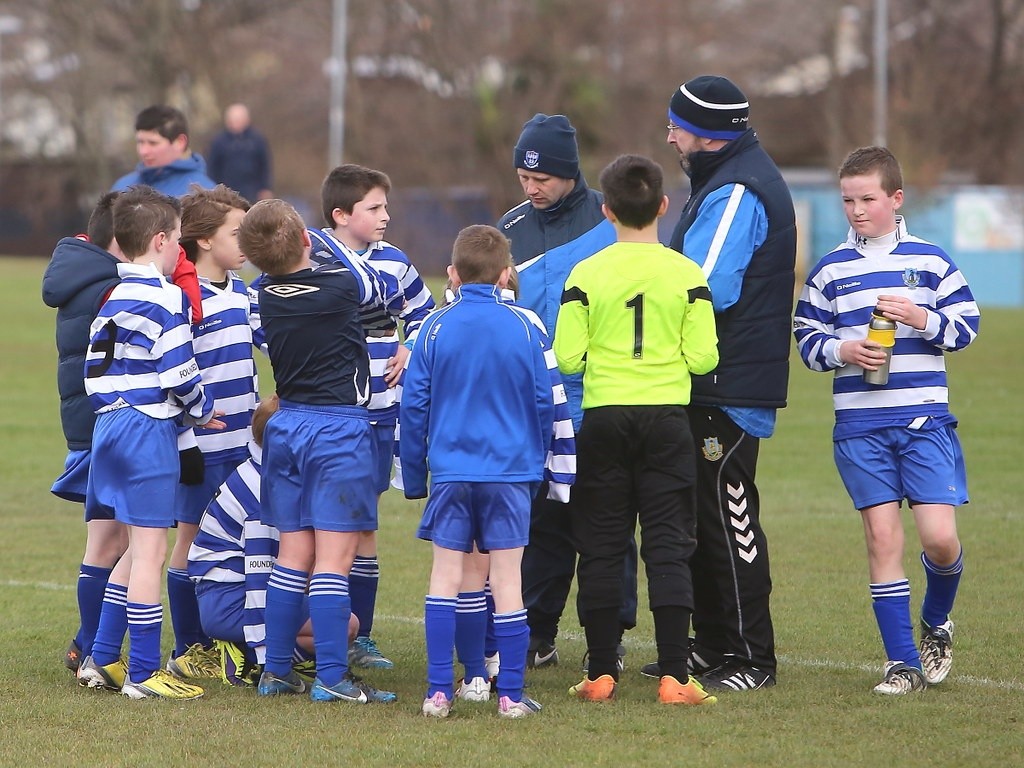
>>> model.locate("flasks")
[862,307,897,386]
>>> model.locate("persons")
[187,396,361,693]
[168,183,315,678]
[794,146,979,693]
[238,200,395,700]
[204,104,272,202]
[639,76,798,692]
[77,185,225,699]
[111,104,216,198]
[43,192,130,670]
[402,222,577,718]
[496,113,624,669]
[246,166,435,666]
[551,155,718,703]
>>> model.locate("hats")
[668,76,750,140]
[512,113,580,180]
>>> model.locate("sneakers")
[483,650,501,681]
[567,673,617,703]
[581,645,627,677]
[454,677,492,702]
[64,637,398,703]
[526,642,558,669]
[698,667,777,691]
[423,691,453,718]
[873,658,928,696]
[642,634,722,677]
[917,613,954,685]
[497,696,542,720]
[658,673,718,706]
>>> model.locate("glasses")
[667,125,679,133]
